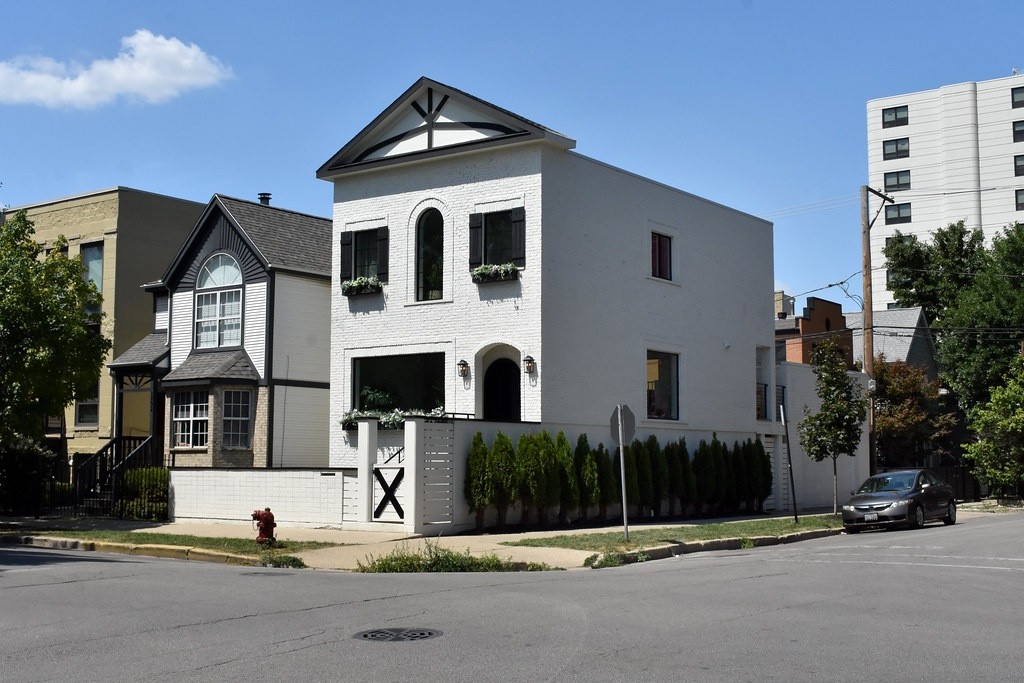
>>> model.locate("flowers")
[338,406,448,431]
[341,275,382,296]
[470,262,517,283]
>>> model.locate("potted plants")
[425,261,443,299]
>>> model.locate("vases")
[472,270,517,282]
[342,285,381,295]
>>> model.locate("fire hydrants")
[251,507,276,544]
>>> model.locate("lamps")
[457,359,468,377]
[523,356,534,373]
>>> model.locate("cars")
[841,469,956,534]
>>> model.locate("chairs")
[908,479,914,487]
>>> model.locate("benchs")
[894,479,927,489]
